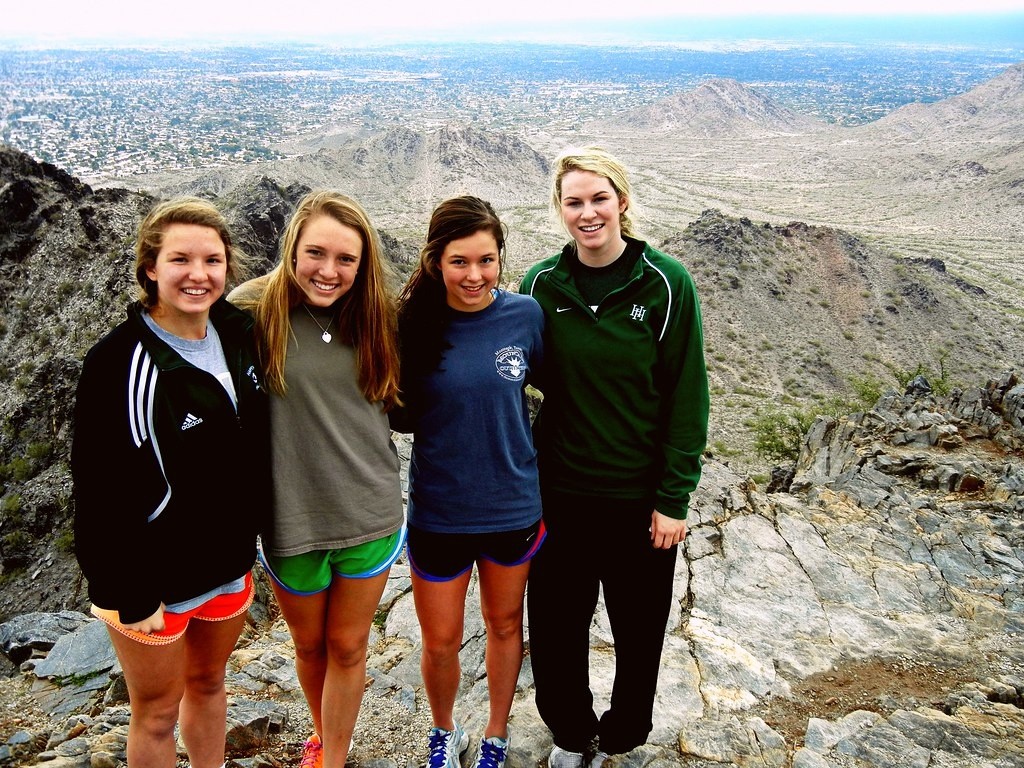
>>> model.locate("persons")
[518,153,709,768]
[70,200,273,768]
[224,186,409,768]
[386,193,547,768]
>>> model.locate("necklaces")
[301,302,336,344]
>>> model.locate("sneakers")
[426,717,470,768]
[472,731,509,768]
[299,732,323,768]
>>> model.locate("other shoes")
[550,744,584,768]
[592,752,609,768]
